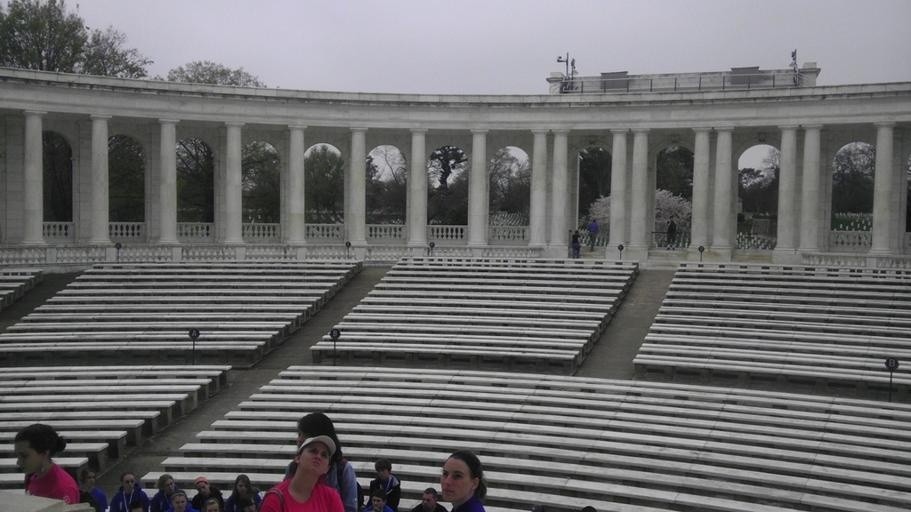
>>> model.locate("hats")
[192,476,208,485]
[297,435,336,457]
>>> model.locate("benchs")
[1,256,911,510]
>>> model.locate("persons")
[571,230,582,258]
[73,466,262,511]
[10,423,81,505]
[667,217,676,250]
[587,219,599,252]
[438,451,491,512]
[411,488,447,511]
[357,463,401,511]
[259,435,345,511]
[282,411,361,512]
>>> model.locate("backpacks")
[288,458,364,508]
